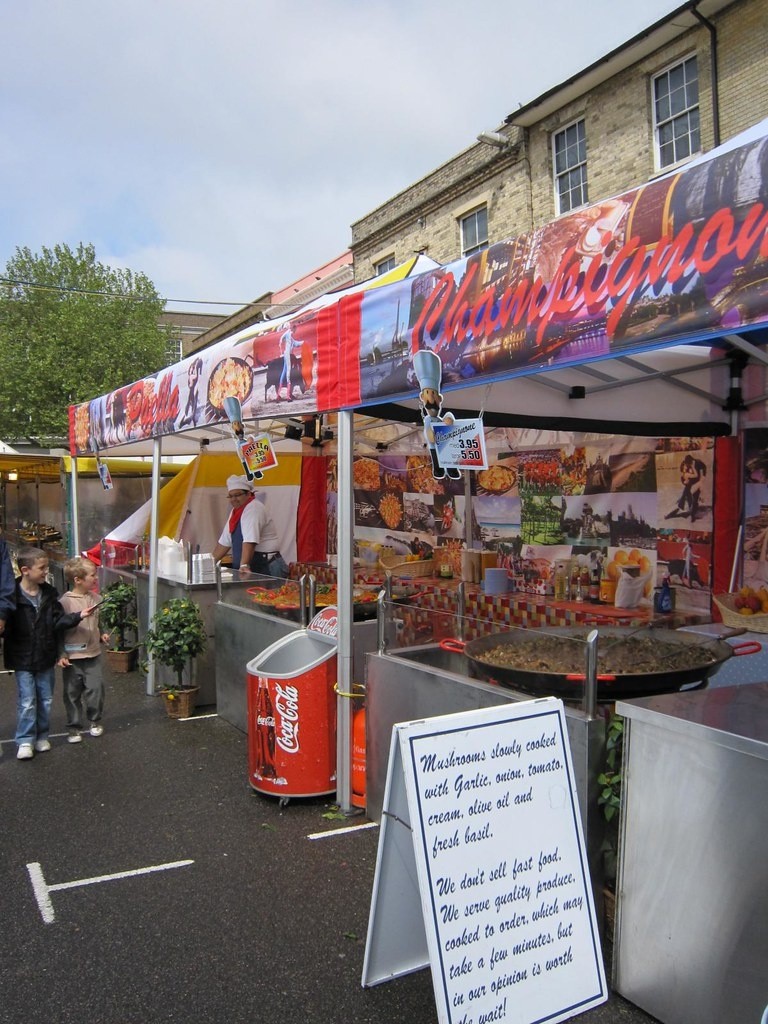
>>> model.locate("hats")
[227,474,254,492]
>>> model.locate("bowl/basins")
[477,465,517,493]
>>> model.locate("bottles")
[566,561,603,605]
[555,564,567,601]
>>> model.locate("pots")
[440,626,762,700]
[246,581,426,623]
[207,355,256,415]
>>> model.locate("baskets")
[378,555,436,578]
[711,588,768,633]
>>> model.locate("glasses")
[227,491,248,500]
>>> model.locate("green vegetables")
[313,585,330,595]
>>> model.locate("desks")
[291,560,712,648]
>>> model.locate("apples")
[735,596,762,612]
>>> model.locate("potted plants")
[147,597,207,718]
[596,713,625,937]
[99,575,138,673]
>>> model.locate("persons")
[665,455,708,522]
[0,538,18,634]
[518,448,588,494]
[3,546,98,760]
[9,483,39,526]
[212,475,290,589]
[56,557,111,743]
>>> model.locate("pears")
[738,583,768,615]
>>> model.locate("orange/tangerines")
[606,549,653,598]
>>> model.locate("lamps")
[8,468,20,483]
[476,131,508,150]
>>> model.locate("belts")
[268,554,279,564]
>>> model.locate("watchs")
[241,564,251,568]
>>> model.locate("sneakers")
[67,735,82,743]
[89,724,103,737]
[17,743,33,759]
[35,740,50,751]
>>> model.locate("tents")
[65,252,726,806]
[342,118,768,812]
